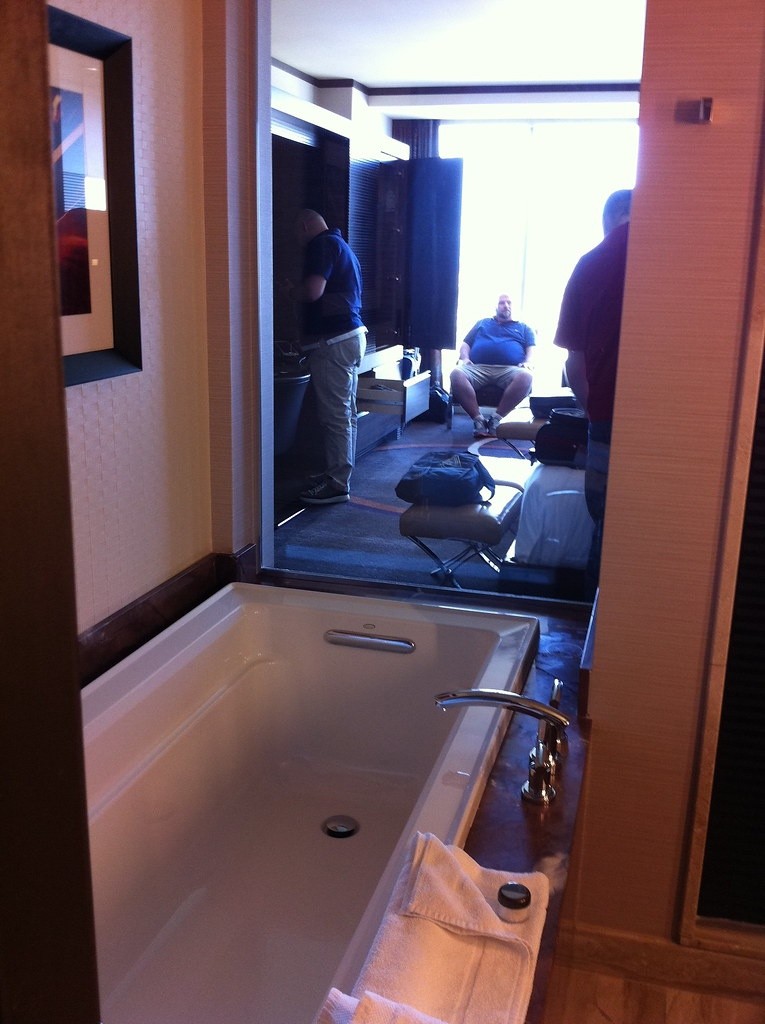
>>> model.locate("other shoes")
[472,415,488,436]
[487,416,499,437]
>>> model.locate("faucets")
[431,677,571,810]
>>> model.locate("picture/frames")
[47,4,144,387]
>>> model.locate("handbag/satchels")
[395,451,495,506]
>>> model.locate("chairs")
[450,383,505,425]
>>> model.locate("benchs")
[395,450,533,595]
[496,386,573,458]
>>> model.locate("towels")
[318,830,551,1023]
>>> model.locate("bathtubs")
[82,580,541,1023]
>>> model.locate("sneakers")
[307,477,333,487]
[299,480,351,504]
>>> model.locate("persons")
[450,294,536,438]
[553,190,633,527]
[283,209,368,504]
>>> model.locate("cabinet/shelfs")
[278,343,430,500]
